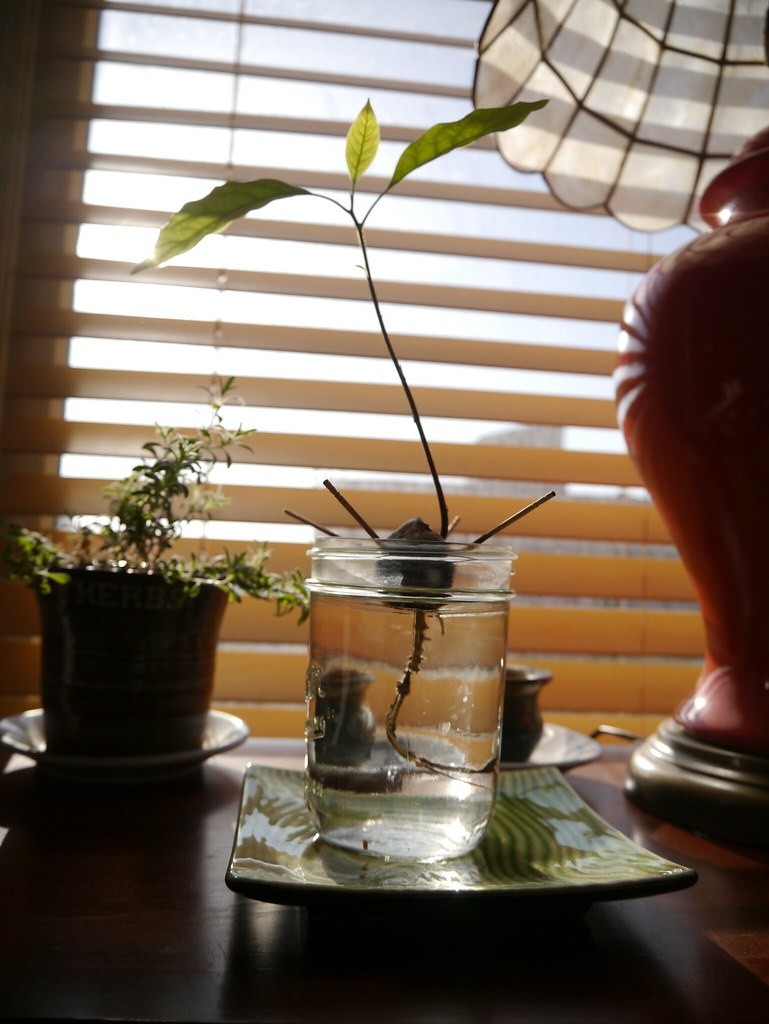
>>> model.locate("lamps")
[473,1,769,751]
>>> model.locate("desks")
[0,736,769,1023]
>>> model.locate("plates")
[2,705,249,784]
[500,723,605,772]
[223,764,700,948]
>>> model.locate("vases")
[304,538,521,862]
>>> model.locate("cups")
[304,535,513,860]
[497,666,554,762]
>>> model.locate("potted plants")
[131,99,552,788]
[1,376,312,784]
